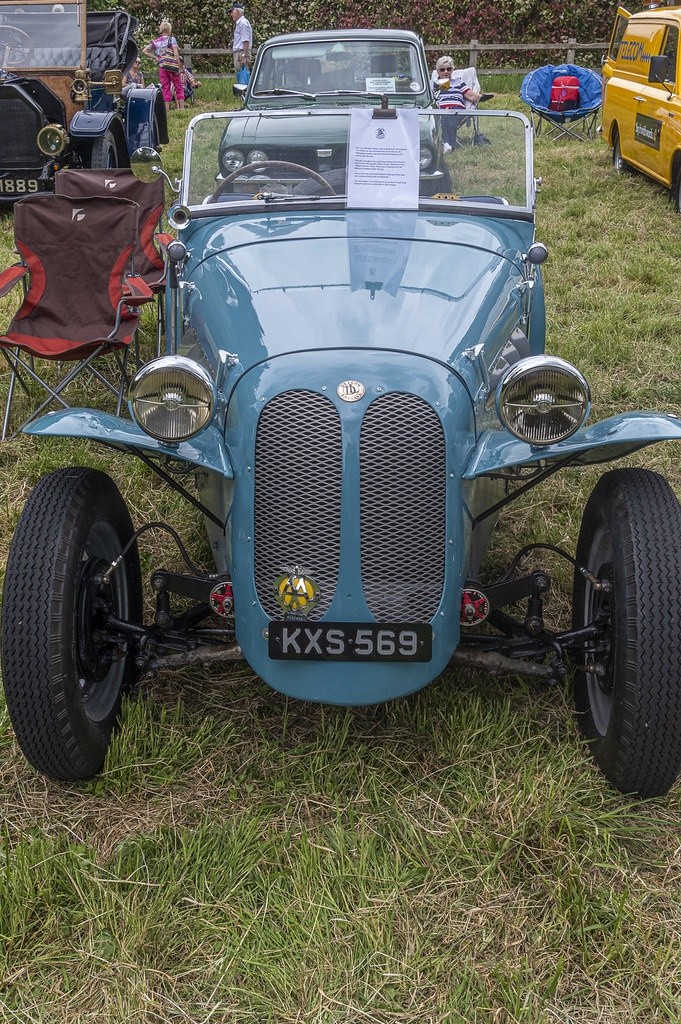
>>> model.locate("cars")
[0,108,681,801]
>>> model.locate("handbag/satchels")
[473,134,492,145]
[158,50,183,73]
[236,65,250,85]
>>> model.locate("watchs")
[242,54,246,57]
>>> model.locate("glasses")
[135,61,141,64]
[440,67,451,72]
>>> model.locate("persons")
[142,22,185,110]
[171,56,202,103]
[120,57,145,89]
[227,3,253,111]
[433,56,494,155]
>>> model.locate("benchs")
[0,46,120,80]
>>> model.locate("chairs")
[292,59,321,85]
[371,53,397,78]
[520,63,604,143]
[154,67,200,105]
[428,67,494,146]
[0,168,174,444]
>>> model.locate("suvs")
[0,0,171,215]
[216,28,451,199]
[599,5,681,210]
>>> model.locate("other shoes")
[442,143,452,156]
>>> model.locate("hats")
[179,56,184,63]
[228,3,242,12]
